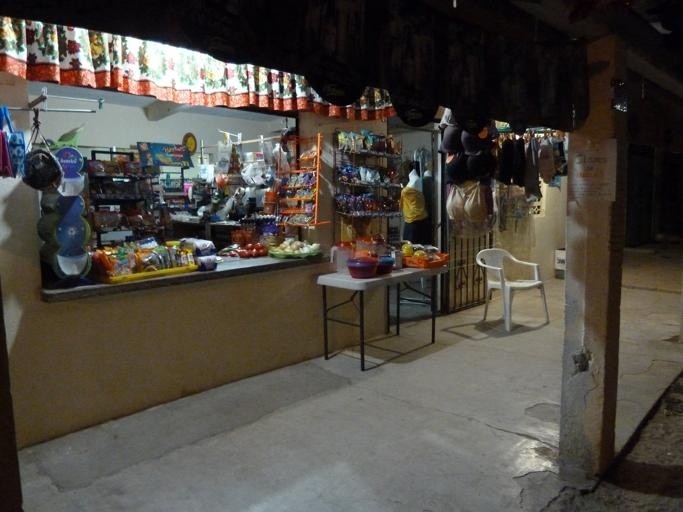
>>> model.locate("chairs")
[476,248,550,332]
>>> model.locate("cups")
[329,241,352,275]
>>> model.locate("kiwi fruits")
[279,239,312,250]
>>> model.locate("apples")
[228,242,268,257]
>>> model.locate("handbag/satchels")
[0,107,15,177]
[3,106,25,178]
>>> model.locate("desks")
[317,265,449,372]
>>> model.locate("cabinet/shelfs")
[85,135,299,251]
[330,128,403,243]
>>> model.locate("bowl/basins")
[347,257,394,278]
